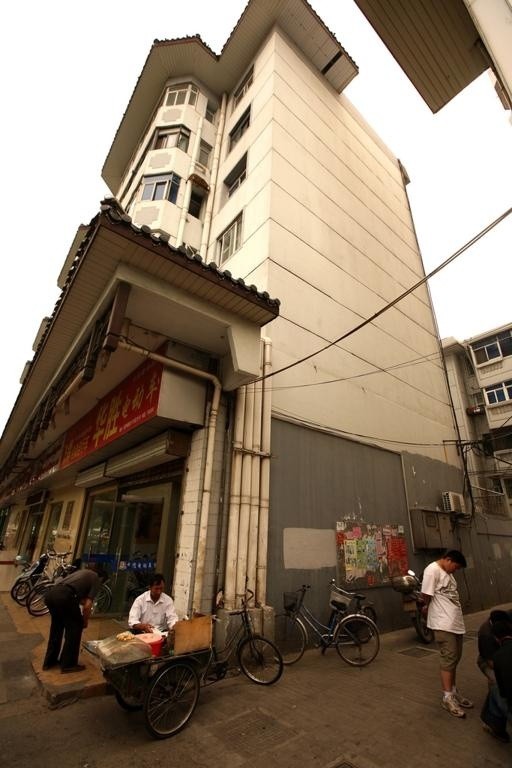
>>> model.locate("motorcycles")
[391,569,434,644]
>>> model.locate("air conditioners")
[442,492,466,513]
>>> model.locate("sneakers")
[42,656,86,675]
[440,692,466,718]
[453,685,473,708]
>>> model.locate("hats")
[445,549,467,569]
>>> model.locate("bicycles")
[273,578,380,667]
[11,543,112,618]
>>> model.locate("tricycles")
[81,589,284,740]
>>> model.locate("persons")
[127,573,180,634]
[42,564,108,674]
[420,549,474,719]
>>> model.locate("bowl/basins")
[147,638,165,657]
